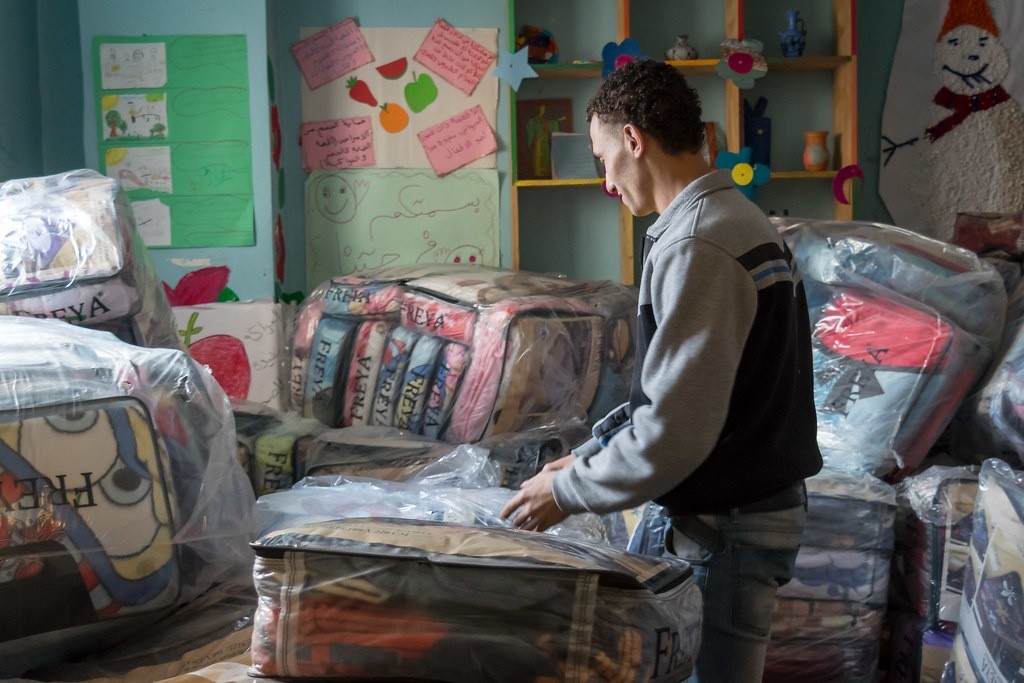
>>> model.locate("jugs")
[781,9,807,57]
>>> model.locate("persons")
[500,61,824,683]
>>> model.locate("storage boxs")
[168,300,295,412]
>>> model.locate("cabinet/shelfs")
[505,0,858,289]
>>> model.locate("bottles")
[802,130,830,171]
